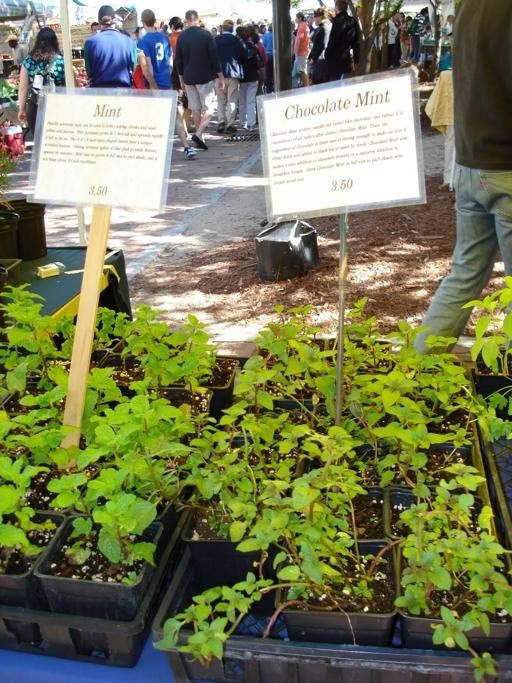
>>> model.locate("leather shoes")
[191,134,208,149]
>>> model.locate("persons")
[3,34,29,67]
[89,21,100,36]
[138,0,406,161]
[83,4,138,87]
[18,26,64,143]
[406,0,454,64]
[406,0,511,355]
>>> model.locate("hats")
[99,5,116,21]
[222,19,233,25]
[169,16,181,24]
[5,34,18,42]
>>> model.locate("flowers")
[0,120,25,211]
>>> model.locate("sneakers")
[238,122,247,128]
[226,126,236,134]
[247,124,255,131]
[185,147,197,159]
[217,123,225,132]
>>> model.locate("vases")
[0,198,46,259]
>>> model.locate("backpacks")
[30,57,57,106]
[406,17,423,33]
